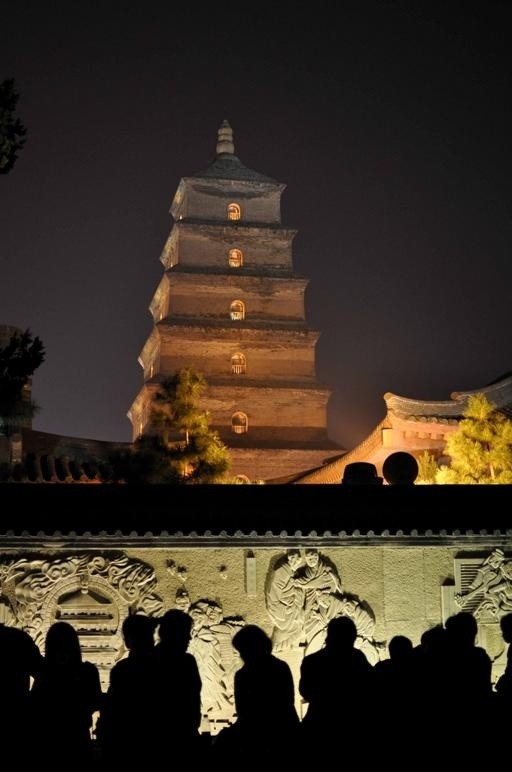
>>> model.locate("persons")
[452,547,512,644]
[1,610,512,771]
[159,547,383,713]
